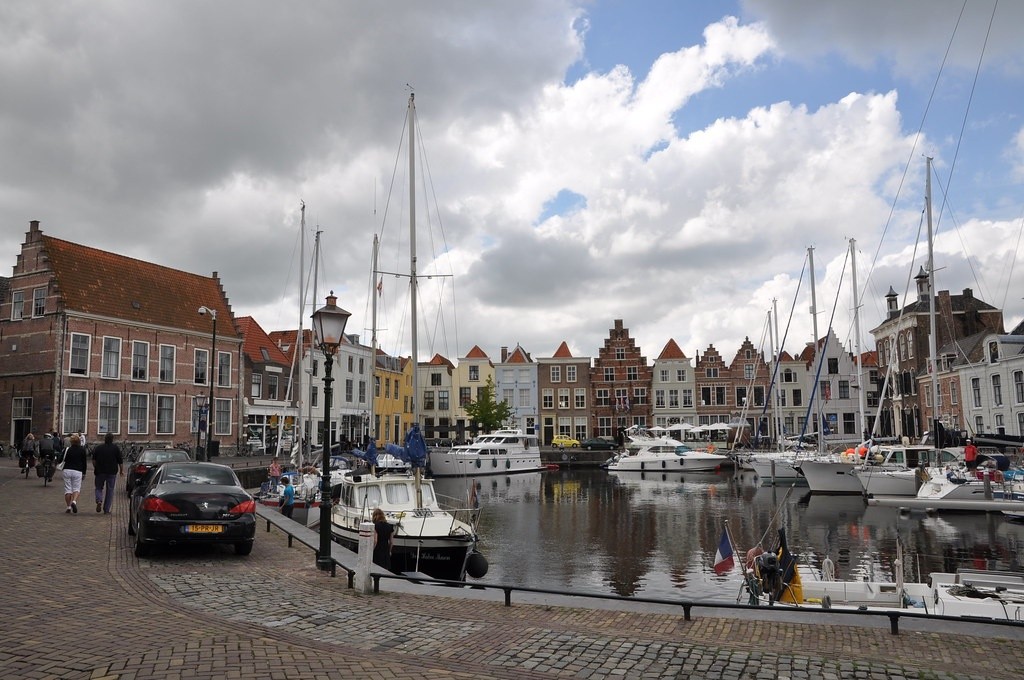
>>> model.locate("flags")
[615,395,631,412]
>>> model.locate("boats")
[913,452,1024,499]
[604,439,727,472]
[429,424,547,475]
[745,566,1024,625]
[992,471,1024,518]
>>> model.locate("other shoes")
[21,469,25,473]
[96,502,102,512]
[66,509,71,513]
[71,502,78,513]
[48,479,52,482]
[104,511,111,514]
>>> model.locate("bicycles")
[225,442,249,457]
[36,454,56,487]
[86,439,194,461]
[25,458,30,479]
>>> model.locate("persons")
[92,433,124,514]
[269,457,282,494]
[964,439,978,471]
[372,509,394,571]
[280,477,294,520]
[19,430,86,482]
[552,442,564,452]
[57,435,87,514]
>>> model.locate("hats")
[44,433,51,437]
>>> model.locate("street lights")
[309,290,352,571]
[194,391,207,462]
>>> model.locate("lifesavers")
[708,446,714,453]
[994,470,1003,483]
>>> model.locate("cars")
[125,448,191,497]
[550,435,580,449]
[128,461,255,558]
[581,437,620,450]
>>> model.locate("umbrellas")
[624,422,732,433]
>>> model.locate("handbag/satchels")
[56,462,65,471]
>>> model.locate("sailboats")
[793,237,891,494]
[726,245,844,480]
[331,92,483,575]
[249,203,352,529]
[853,154,1010,497]
